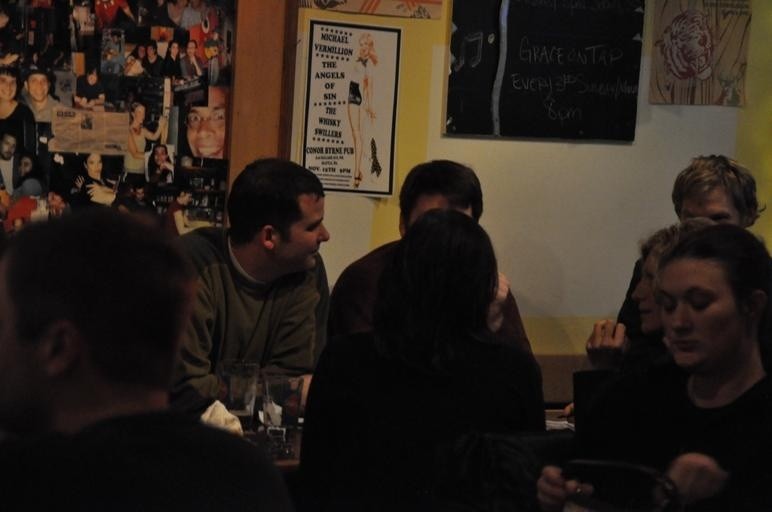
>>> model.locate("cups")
[264,374,305,449]
[227,360,260,429]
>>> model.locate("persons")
[344,30,379,187]
[291,206,546,512]
[671,145,762,236]
[2,193,280,508]
[0,0,233,240]
[568,225,677,452]
[532,223,772,512]
[146,155,332,464]
[322,154,529,344]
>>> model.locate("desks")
[278,415,578,473]
